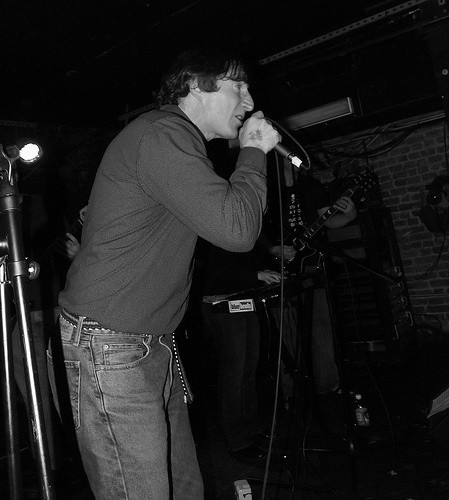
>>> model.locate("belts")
[60,306,194,403]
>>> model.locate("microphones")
[274,143,306,169]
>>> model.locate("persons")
[260,141,358,410]
[59,47,282,500]
[420,175,449,235]
[0,186,89,469]
[198,237,264,461]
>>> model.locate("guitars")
[247,169,365,290]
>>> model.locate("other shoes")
[50,451,60,470]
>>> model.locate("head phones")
[427,176,449,205]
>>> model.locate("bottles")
[352,393,368,427]
